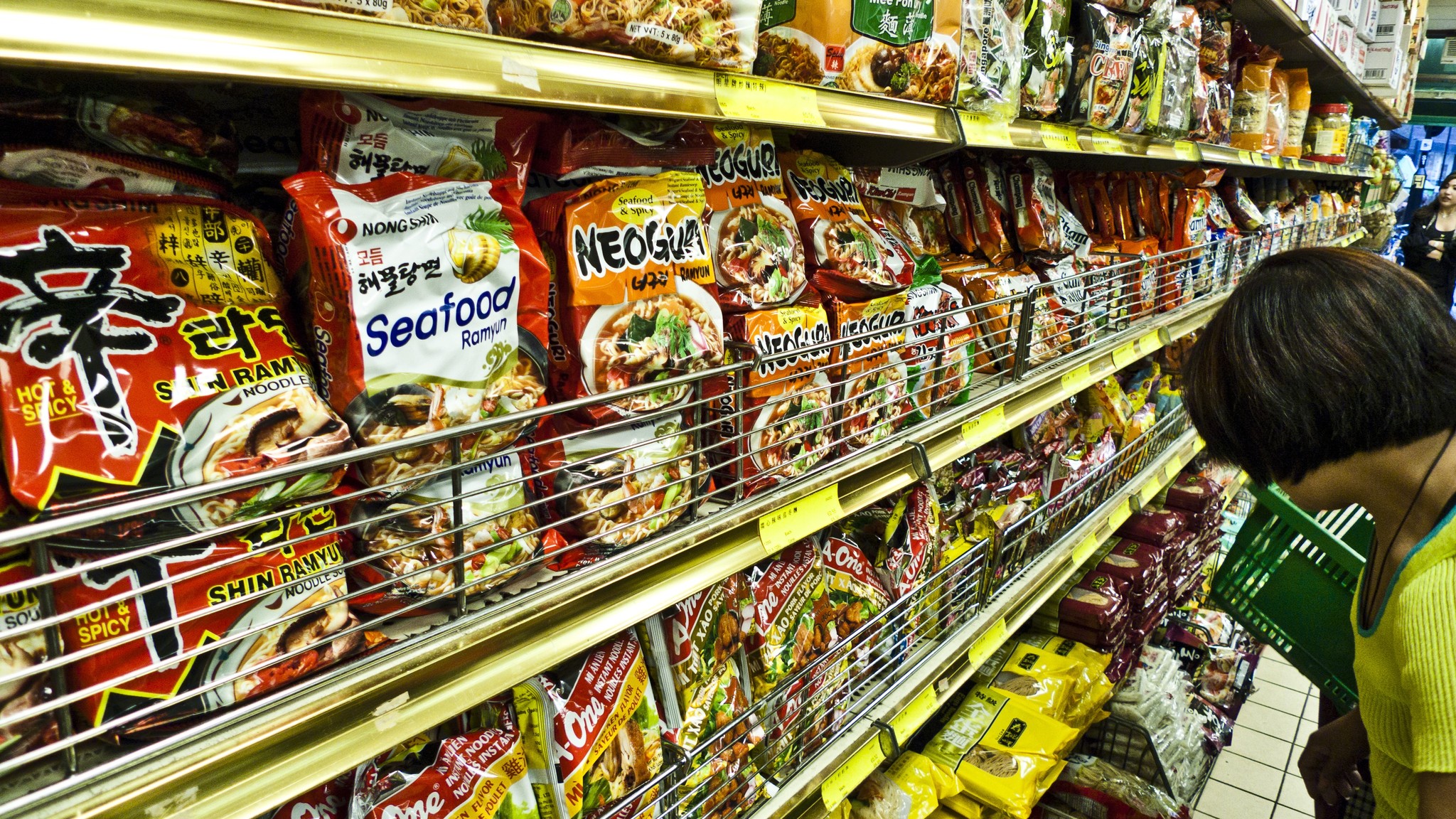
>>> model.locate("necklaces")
[1437,207,1456,218]
[1361,432,1454,629]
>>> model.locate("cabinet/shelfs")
[0,0,1426,818]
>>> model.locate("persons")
[1177,245,1456,819]
[1402,171,1456,316]
